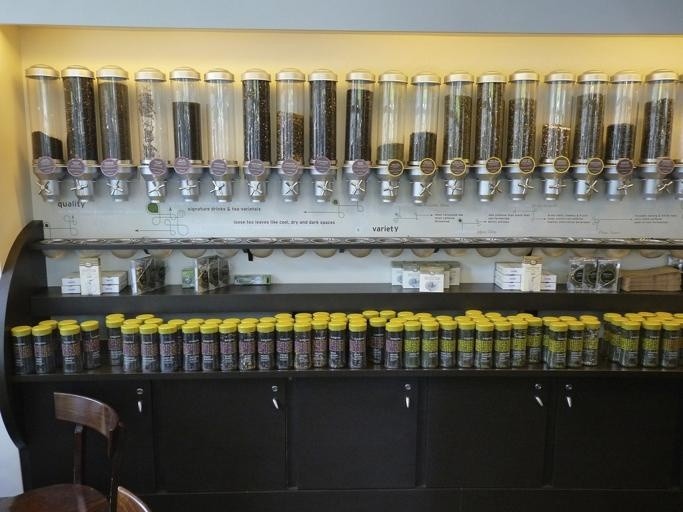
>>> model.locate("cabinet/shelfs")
[423,371,683,493]
[23,231,682,316]
[289,376,425,491]
[153,378,289,490]
[12,377,155,489]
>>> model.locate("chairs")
[0,390,124,512]
[115,476,153,512]
